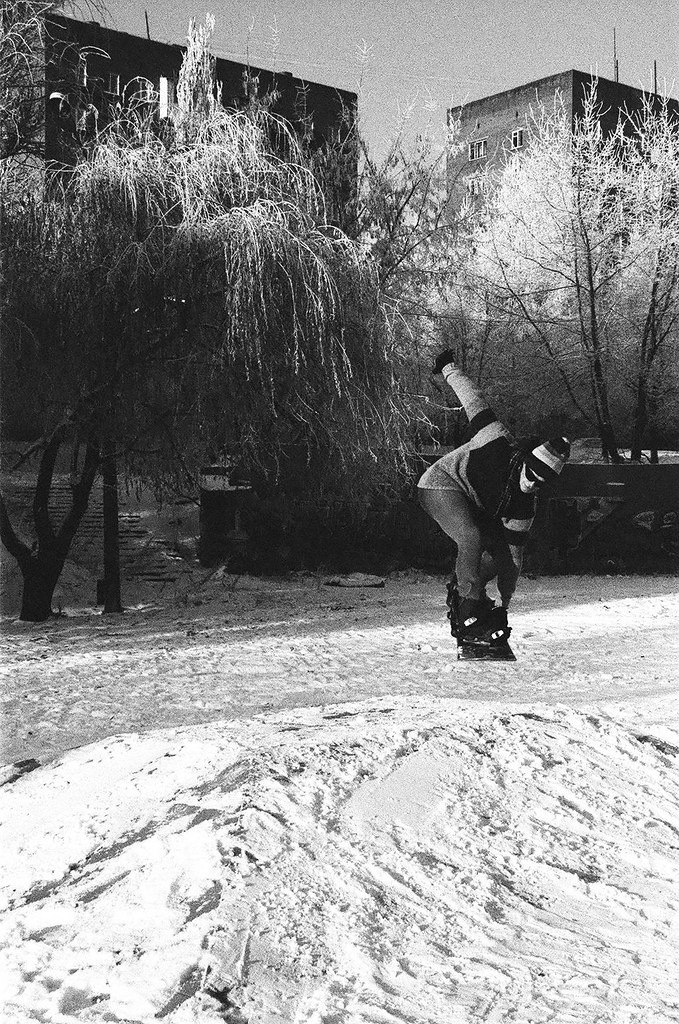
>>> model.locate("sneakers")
[446,576,511,644]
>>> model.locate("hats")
[527,437,571,482]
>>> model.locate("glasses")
[525,467,545,488]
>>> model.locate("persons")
[416,349,571,659]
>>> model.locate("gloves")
[432,349,455,374]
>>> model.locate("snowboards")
[446,581,518,660]
